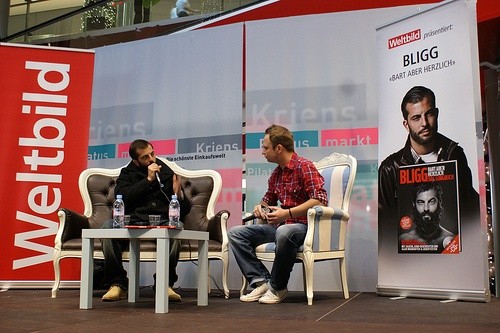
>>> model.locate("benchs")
[51,153,231,299]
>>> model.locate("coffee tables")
[79,228,209,313]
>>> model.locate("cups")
[149,215,161,226]
[124,215,130,225]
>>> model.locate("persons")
[227,124,328,303]
[133,0,150,25]
[378,85,481,234]
[99,139,191,302]
[175,0,199,18]
[400,181,454,249]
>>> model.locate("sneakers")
[101,286,127,300]
[240,281,270,302]
[168,287,181,301]
[259,288,288,304]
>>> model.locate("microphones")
[242,208,271,223]
[155,172,161,184]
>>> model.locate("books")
[397,159,463,254]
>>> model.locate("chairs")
[239,152,358,306]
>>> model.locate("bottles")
[113,195,125,229]
[168,195,180,227]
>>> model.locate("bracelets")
[288,208,293,217]
